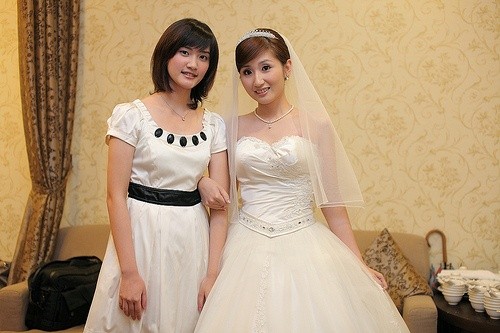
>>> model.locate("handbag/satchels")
[25,255,102,331]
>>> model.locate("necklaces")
[254,104,293,129]
[159,93,192,121]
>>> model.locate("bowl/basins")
[437,277,500,319]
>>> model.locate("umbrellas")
[426,229,454,290]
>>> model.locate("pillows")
[362,228,434,316]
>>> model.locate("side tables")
[432,288,500,333]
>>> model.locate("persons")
[82,18,229,333]
[196,28,410,333]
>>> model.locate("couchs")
[0,225,438,333]
